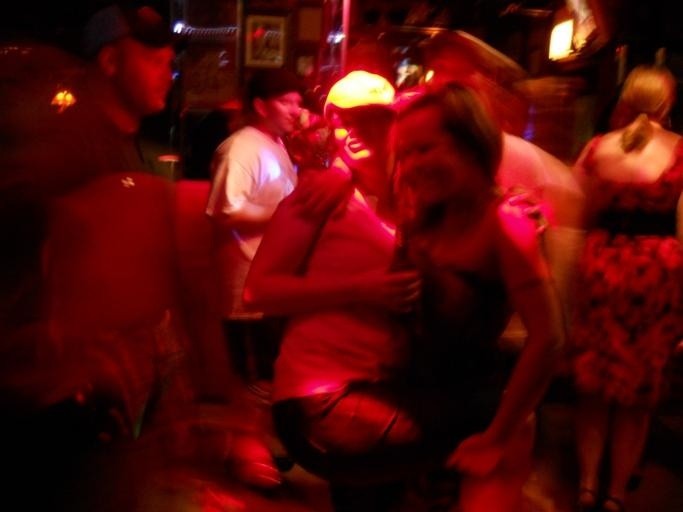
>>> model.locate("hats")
[318,70,396,124]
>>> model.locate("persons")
[1,1,683,511]
